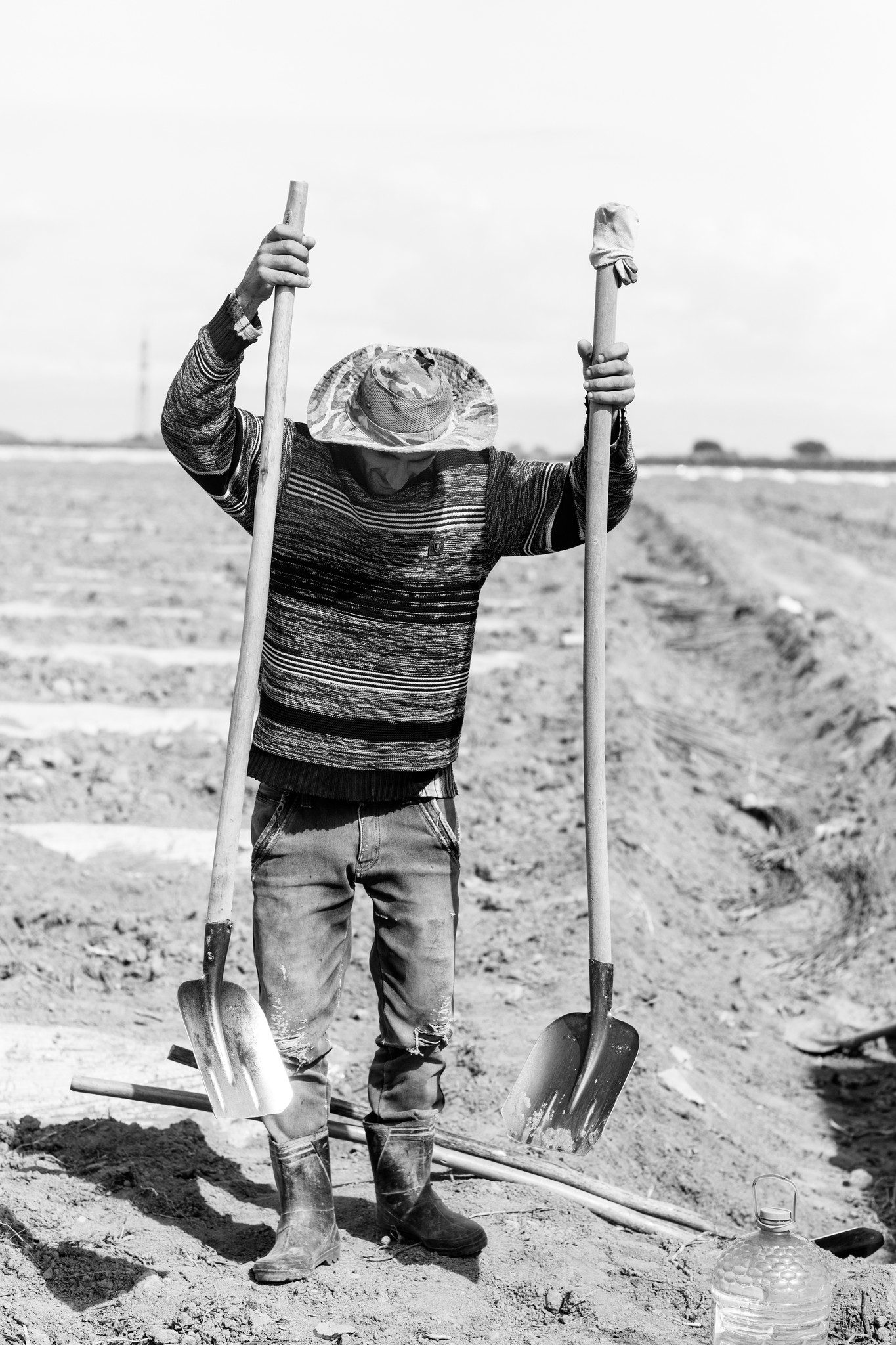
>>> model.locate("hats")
[309,344,499,454]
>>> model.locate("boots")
[252,1129,341,1280]
[363,1109,486,1256]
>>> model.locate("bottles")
[709,1173,833,1344]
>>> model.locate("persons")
[159,222,638,1284]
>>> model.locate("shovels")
[177,177,296,1123]
[498,203,641,1161]
[68,1043,887,1261]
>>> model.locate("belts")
[258,782,387,812]
